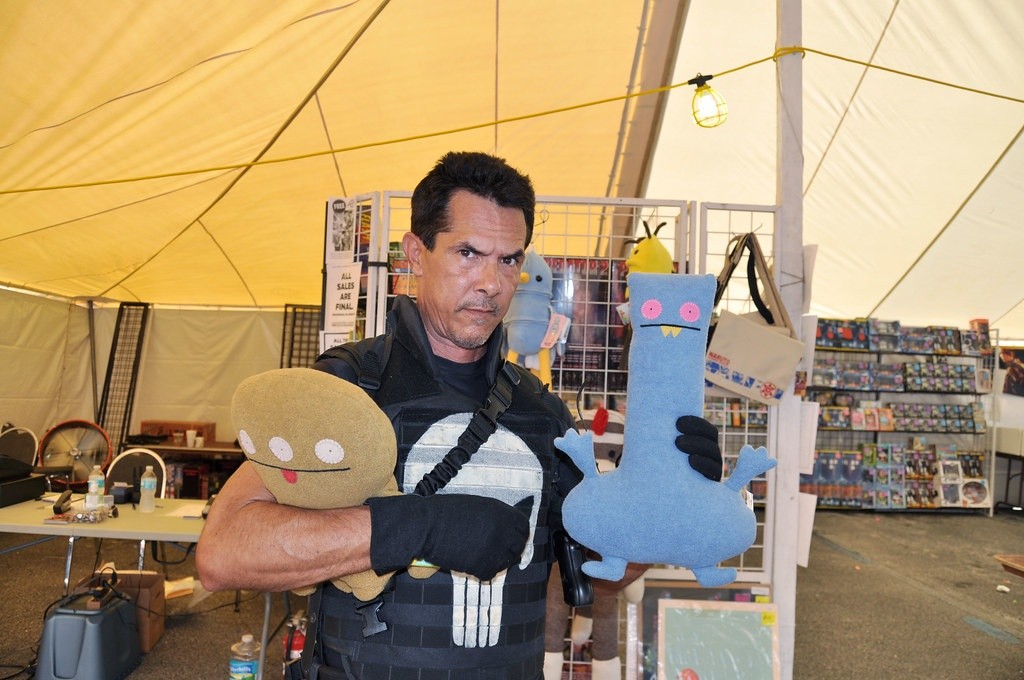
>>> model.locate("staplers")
[53,490,73,514]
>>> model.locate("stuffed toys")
[548,272,777,586]
[231,368,442,602]
[541,400,644,680]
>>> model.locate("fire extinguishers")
[281,610,309,680]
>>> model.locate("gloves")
[362,495,529,581]
[675,414,723,482]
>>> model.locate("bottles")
[139,465,157,513]
[228,632,262,680]
[87,464,106,511]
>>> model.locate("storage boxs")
[74,573,165,653]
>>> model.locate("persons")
[193,146,725,680]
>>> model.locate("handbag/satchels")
[709,232,805,406]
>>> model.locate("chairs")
[61,448,167,598]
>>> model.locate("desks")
[127,438,243,454]
[0,494,209,543]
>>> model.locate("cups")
[186,430,196,448]
[174,433,183,448]
[195,437,204,448]
